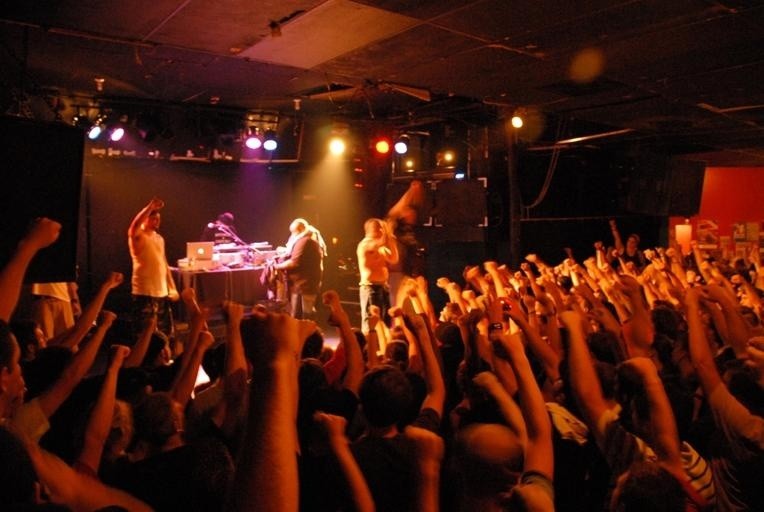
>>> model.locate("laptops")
[177,241,214,263]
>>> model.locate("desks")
[176,258,274,320]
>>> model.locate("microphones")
[207,223,222,229]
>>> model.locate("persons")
[127,196,182,319]
[0,216,764,511]
[389,181,428,308]
